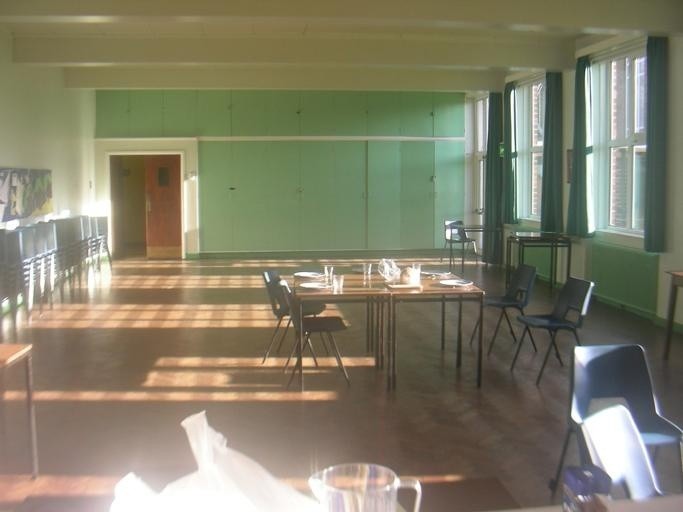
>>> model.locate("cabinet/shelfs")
[197,90,266,138]
[267,141,333,260]
[332,141,401,259]
[93,88,162,138]
[400,140,466,259]
[266,89,332,138]
[331,89,401,138]
[162,89,197,138]
[401,90,466,137]
[197,141,267,259]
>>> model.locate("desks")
[451,224,502,271]
[506,234,571,298]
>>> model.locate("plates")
[300,282,330,289]
[440,279,473,286]
[294,271,322,278]
[421,271,451,276]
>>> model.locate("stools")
[0,342,38,480]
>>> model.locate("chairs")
[550,345,683,500]
[512,276,593,386]
[261,271,328,367]
[469,266,537,355]
[280,280,351,387]
[440,219,477,269]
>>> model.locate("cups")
[413,263,421,273]
[333,275,344,293]
[363,263,372,275]
[309,462,422,508]
[325,265,333,277]
